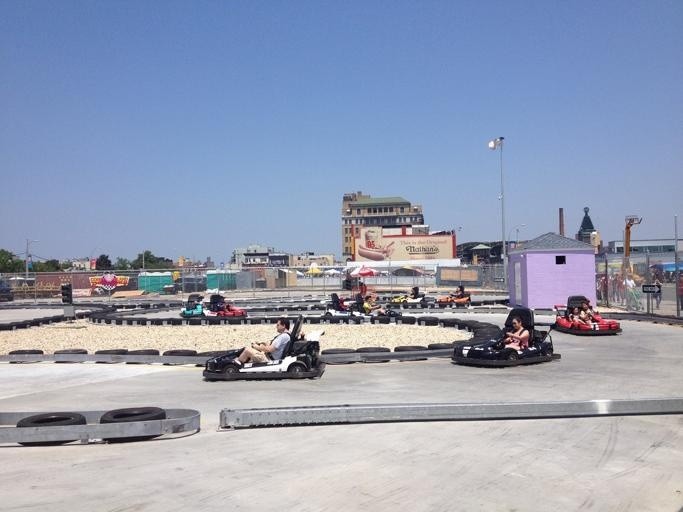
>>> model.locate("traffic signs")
[641,283,660,294]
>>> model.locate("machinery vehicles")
[616,213,645,288]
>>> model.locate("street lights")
[457,225,463,258]
[515,224,527,246]
[488,135,507,286]
[25,239,40,280]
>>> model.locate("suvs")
[163,277,207,294]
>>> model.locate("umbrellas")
[350,265,376,293]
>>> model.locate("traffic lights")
[61,285,72,304]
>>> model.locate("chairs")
[565,296,592,321]
[331,293,343,311]
[251,315,304,367]
[356,294,366,313]
[210,295,221,312]
[499,308,535,348]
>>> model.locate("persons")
[580,300,594,324]
[234,318,292,368]
[192,300,200,308]
[339,297,348,309]
[450,286,464,297]
[408,287,418,299]
[217,301,229,312]
[596,265,683,311]
[362,295,385,316]
[568,306,585,325]
[503,316,532,350]
